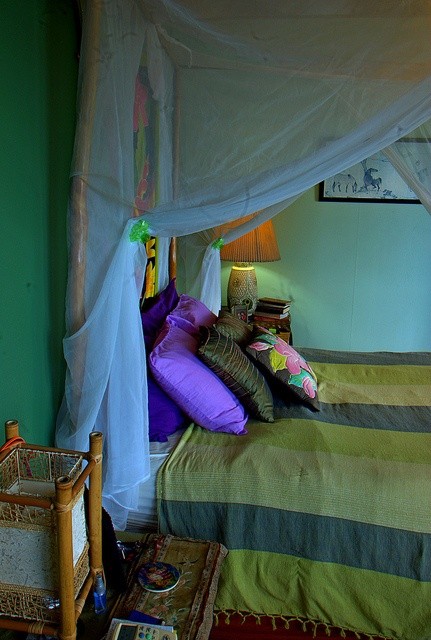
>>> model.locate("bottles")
[94,575,106,615]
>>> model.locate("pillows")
[198,324,276,424]
[149,294,250,437]
[212,309,253,346]
[140,277,190,444]
[245,323,321,413]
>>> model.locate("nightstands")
[222,305,292,345]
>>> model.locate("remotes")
[111,623,178,640]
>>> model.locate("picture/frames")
[317,134,431,205]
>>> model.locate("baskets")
[0,448,90,625]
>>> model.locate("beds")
[62,1,431,640]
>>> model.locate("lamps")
[208,206,282,317]
[0,419,109,640]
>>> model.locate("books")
[253,297,292,322]
